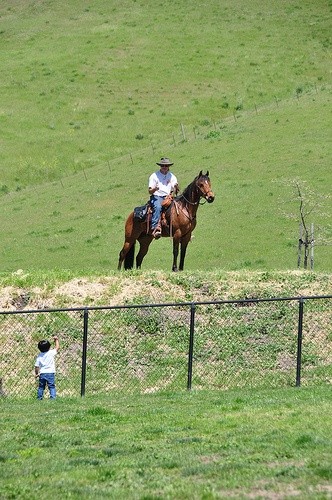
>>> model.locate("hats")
[156,157,174,166]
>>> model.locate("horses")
[118,170,214,271]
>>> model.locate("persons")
[34,335,60,400]
[148,157,178,239]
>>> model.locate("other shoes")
[154,231,162,240]
